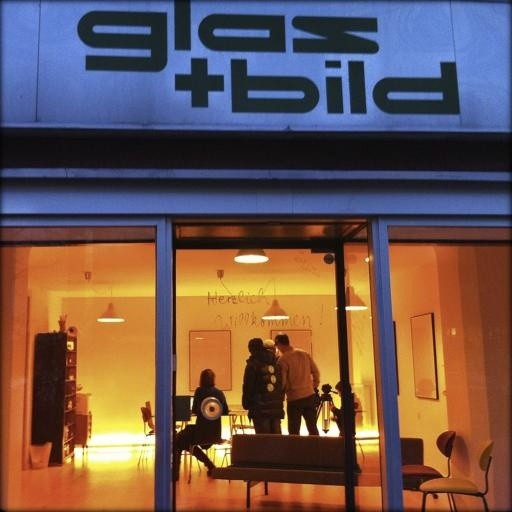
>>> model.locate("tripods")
[317,393,364,457]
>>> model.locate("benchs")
[207,433,360,509]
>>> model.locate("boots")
[193,446,215,474]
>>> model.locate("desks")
[186,408,254,461]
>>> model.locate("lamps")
[216,269,289,320]
[234,249,268,265]
[324,253,369,311]
[84,269,126,323]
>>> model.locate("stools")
[230,423,255,435]
[180,435,232,483]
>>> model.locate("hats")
[274,334,289,344]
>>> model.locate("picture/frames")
[392,320,401,396]
[188,330,233,391]
[410,311,439,400]
[271,329,312,358]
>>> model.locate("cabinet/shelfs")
[74,411,92,452]
[34,331,76,466]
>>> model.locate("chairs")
[145,400,156,465]
[401,430,460,512]
[418,439,495,511]
[138,407,156,465]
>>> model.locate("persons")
[261,340,279,359]
[275,334,319,435]
[242,338,284,434]
[331,381,362,436]
[175,369,228,480]
